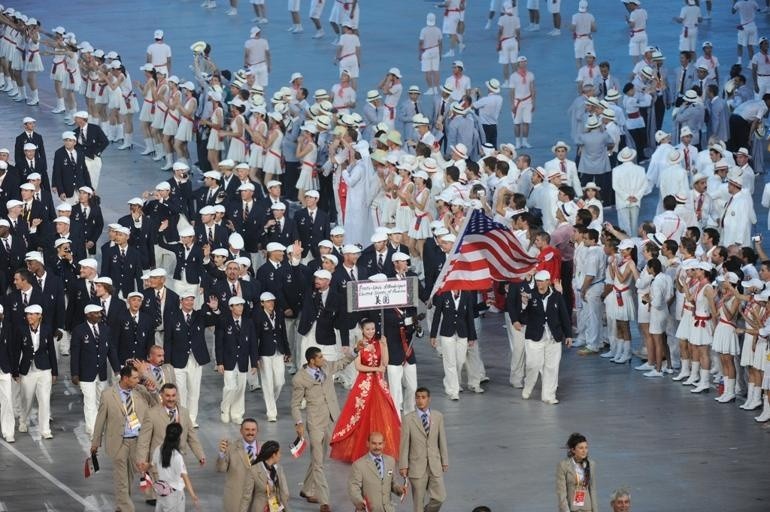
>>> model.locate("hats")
[502,3,515,17]
[453,60,465,72]
[680,40,717,103]
[654,126,752,204]
[3,2,244,121]
[580,0,590,13]
[680,256,769,308]
[342,21,353,30]
[427,12,438,27]
[249,27,259,39]
[534,271,552,282]
[244,182,457,304]
[639,44,666,80]
[757,36,768,45]
[584,50,598,59]
[107,158,242,308]
[246,67,517,181]
[1,130,110,316]
[529,140,602,220]
[615,232,668,250]
[615,146,640,163]
[584,88,623,130]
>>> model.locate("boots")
[610,337,634,366]
[671,358,770,423]
[106,125,171,169]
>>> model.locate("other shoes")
[287,25,303,34]
[442,51,456,59]
[313,28,324,40]
[458,43,466,54]
[251,16,269,25]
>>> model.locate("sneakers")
[2,420,52,443]
[634,360,674,379]
[220,417,244,426]
[446,374,491,401]
[512,380,560,406]
[570,336,610,360]
[266,416,277,422]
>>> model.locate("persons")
[1,1,769,511]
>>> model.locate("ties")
[374,458,383,478]
[314,372,323,380]
[246,448,256,463]
[422,411,429,434]
